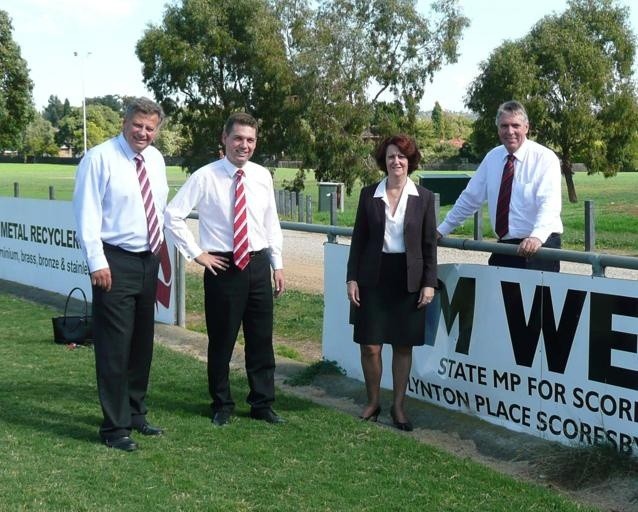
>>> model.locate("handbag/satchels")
[52,316,95,345]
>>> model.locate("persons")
[74,97,170,453]
[164,113,285,428]
[345,134,438,432]
[437,102,564,272]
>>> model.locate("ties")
[135,153,163,256]
[233,170,249,270]
[495,155,515,239]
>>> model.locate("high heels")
[391,406,413,431]
[359,407,380,422]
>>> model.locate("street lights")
[72,50,93,157]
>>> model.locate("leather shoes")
[134,423,163,435]
[213,409,231,425]
[106,436,136,451]
[250,408,289,424]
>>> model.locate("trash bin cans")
[316,181,344,213]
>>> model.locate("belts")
[250,248,271,257]
